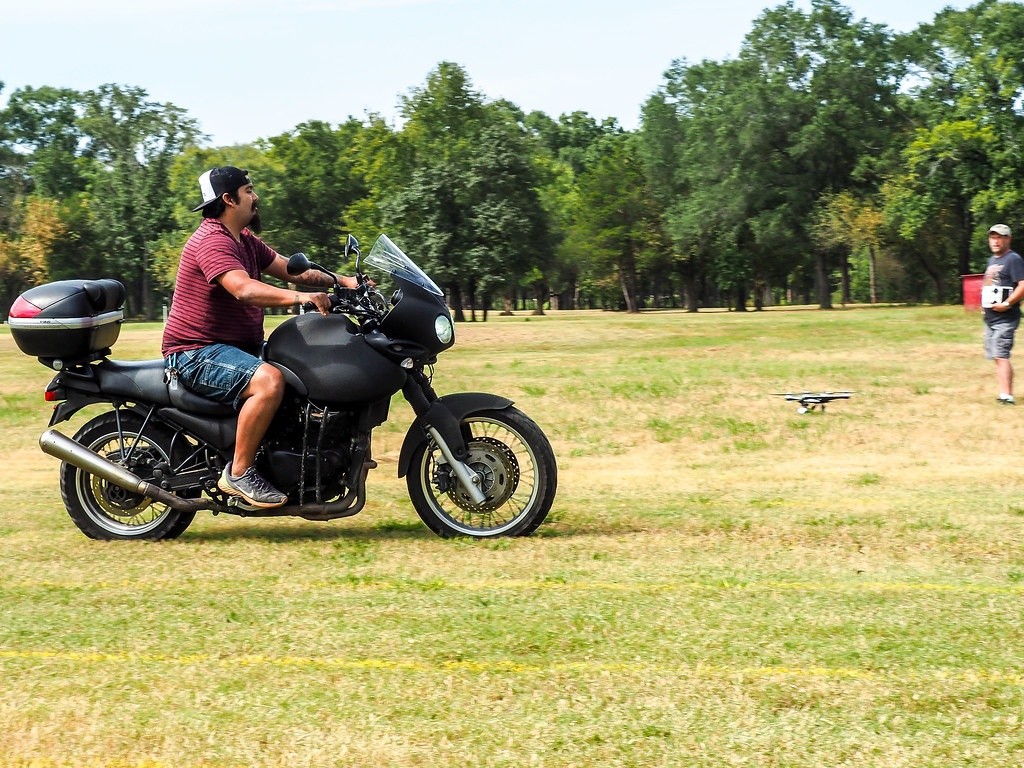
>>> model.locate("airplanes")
[768,390,859,413]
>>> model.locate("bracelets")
[1003,302,1011,308]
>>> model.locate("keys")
[162,367,181,383]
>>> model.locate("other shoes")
[996,393,1015,406]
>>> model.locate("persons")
[160,166,379,509]
[980,224,1024,404]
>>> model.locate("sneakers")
[218,460,288,509]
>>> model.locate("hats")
[987,224,1012,237]
[192,166,251,212]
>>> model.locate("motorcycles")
[6,231,560,543]
